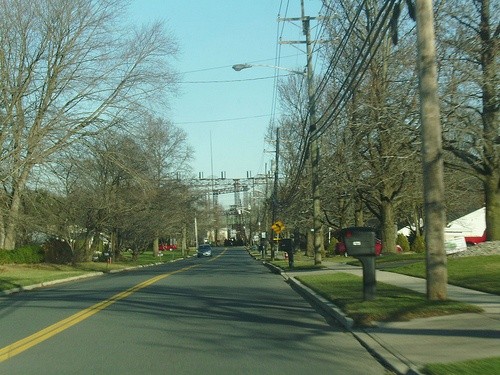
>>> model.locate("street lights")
[232,63,326,266]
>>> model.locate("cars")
[159,242,177,251]
[336,238,404,258]
[196,243,214,257]
